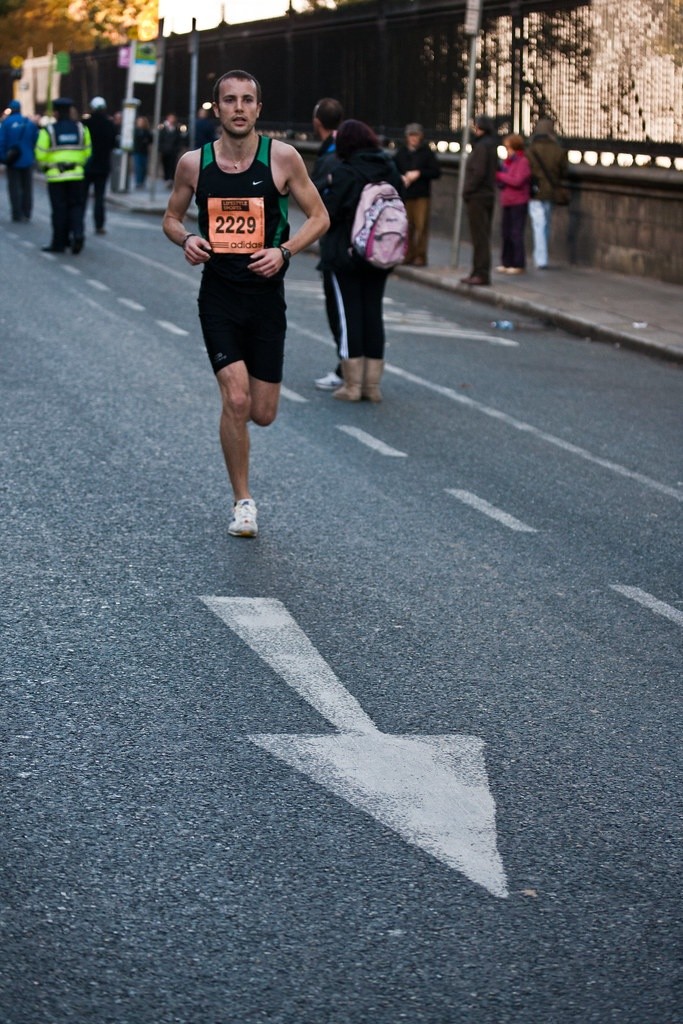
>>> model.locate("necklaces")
[220,137,257,169]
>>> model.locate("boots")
[332,356,363,402]
[363,357,383,402]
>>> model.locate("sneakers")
[227,499,258,537]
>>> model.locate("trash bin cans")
[111,150,135,193]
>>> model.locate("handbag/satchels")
[553,186,574,205]
[4,145,20,165]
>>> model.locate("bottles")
[492,320,512,329]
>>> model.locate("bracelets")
[182,234,196,249]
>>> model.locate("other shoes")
[42,245,63,251]
[316,373,341,390]
[73,239,84,254]
[461,275,487,284]
[499,266,523,274]
[413,256,425,266]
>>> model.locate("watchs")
[280,246,291,264]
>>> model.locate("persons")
[0,100,39,222]
[195,109,216,150]
[496,134,531,275]
[323,119,409,402]
[158,113,189,187]
[460,116,497,285]
[524,119,568,268]
[163,70,330,536]
[77,96,116,235]
[392,123,440,266]
[129,116,152,191]
[311,98,345,390]
[110,112,122,149]
[34,97,92,255]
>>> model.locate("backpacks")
[344,163,410,270]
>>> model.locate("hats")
[52,98,72,110]
[10,101,20,109]
[92,97,107,111]
[406,124,423,136]
